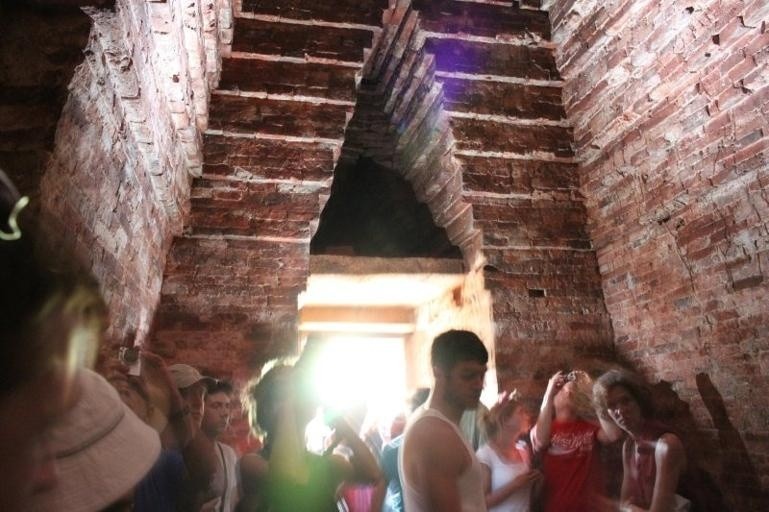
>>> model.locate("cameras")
[109,344,142,377]
[556,371,576,388]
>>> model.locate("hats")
[1,365,162,512]
[167,361,216,391]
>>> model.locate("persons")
[1,204,430,512]
[398,328,692,511]
